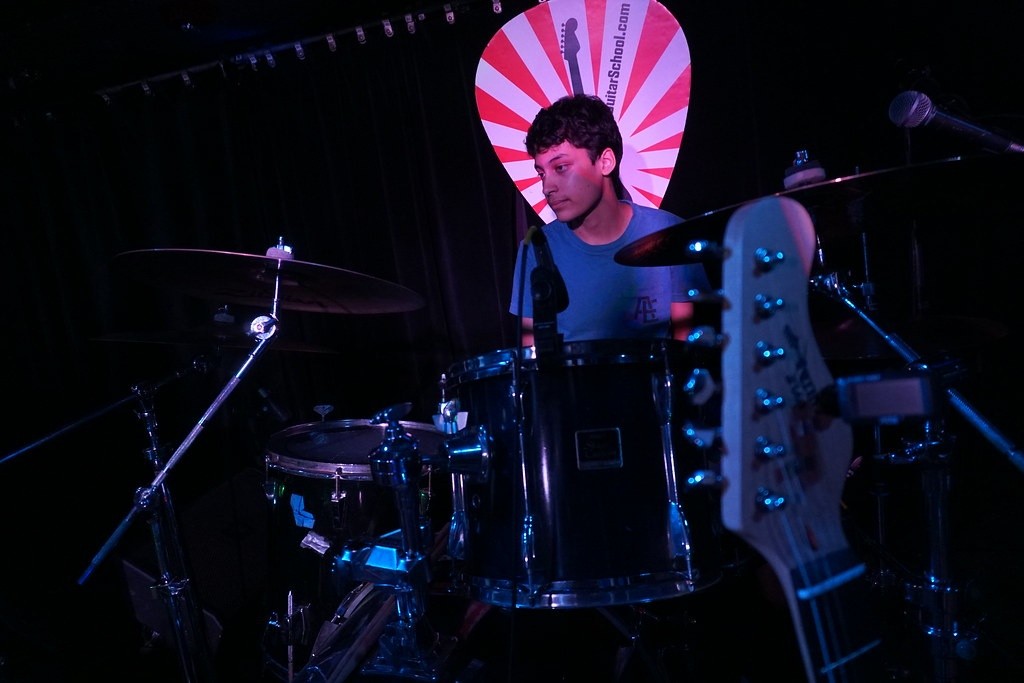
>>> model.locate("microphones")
[888,91,1024,155]
[529,224,568,313]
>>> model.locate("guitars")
[670,188,906,683]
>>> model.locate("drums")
[264,412,457,558]
[435,336,722,616]
[334,581,440,683]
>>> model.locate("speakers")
[120,477,299,661]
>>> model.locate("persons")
[508,94,722,683]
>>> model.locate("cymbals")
[104,234,430,321]
[89,312,347,361]
[612,140,994,271]
